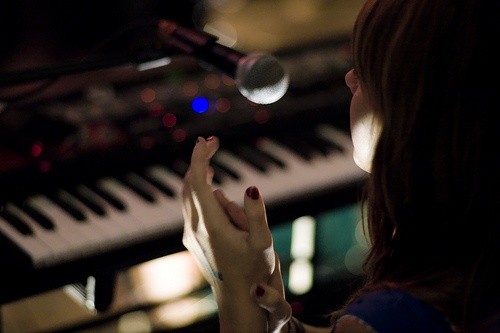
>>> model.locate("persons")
[175,1,499,333]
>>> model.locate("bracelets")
[287,321,293,332]
[290,314,306,332]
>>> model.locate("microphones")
[146,16,289,105]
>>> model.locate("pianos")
[0,28,371,308]
